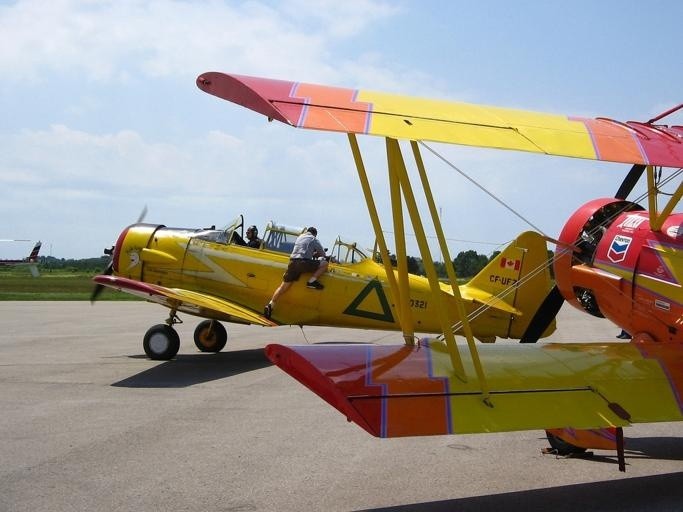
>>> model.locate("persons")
[264,227,330,320]
[246,225,262,246]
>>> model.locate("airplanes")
[0,236,52,279]
[82,199,564,364]
[186,62,683,479]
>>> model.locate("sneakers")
[305,280,324,290]
[263,304,272,319]
